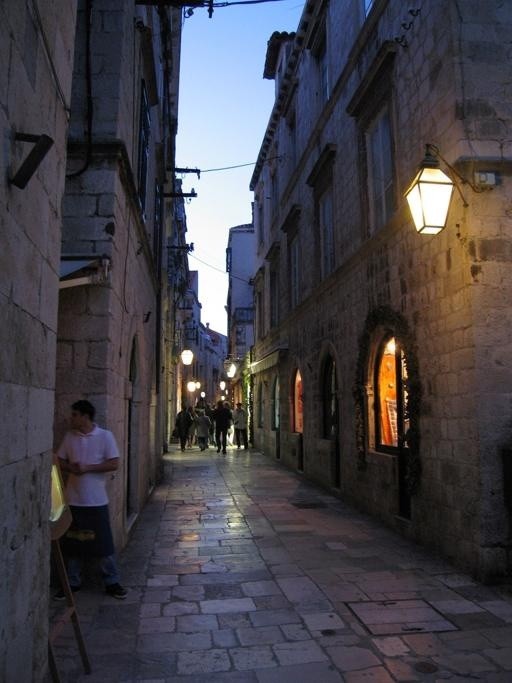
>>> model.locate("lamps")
[400,136,512,239]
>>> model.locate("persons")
[53,400,129,601]
[171,400,248,454]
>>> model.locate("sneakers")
[52,586,80,601]
[106,583,128,598]
